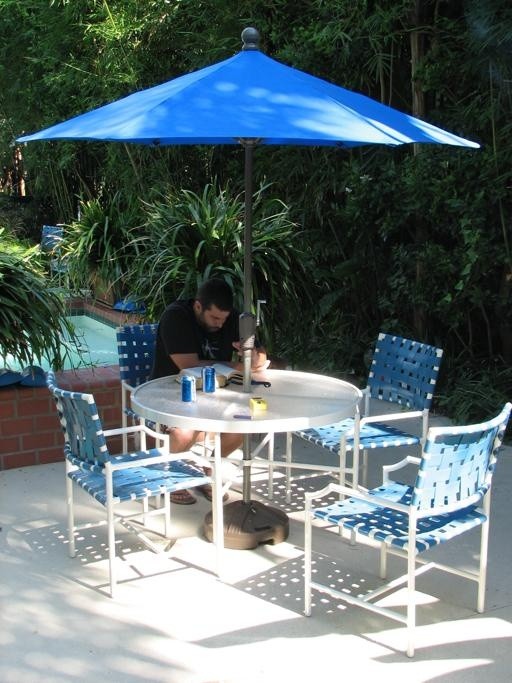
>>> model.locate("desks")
[125,364,362,552]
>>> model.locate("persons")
[150,278,267,505]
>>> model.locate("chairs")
[47,366,219,599]
[283,329,447,503]
[294,403,510,656]
[111,323,169,457]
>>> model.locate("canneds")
[180,375,196,404]
[202,366,216,393]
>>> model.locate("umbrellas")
[16,25,482,504]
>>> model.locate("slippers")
[200,483,228,502]
[162,489,196,504]
